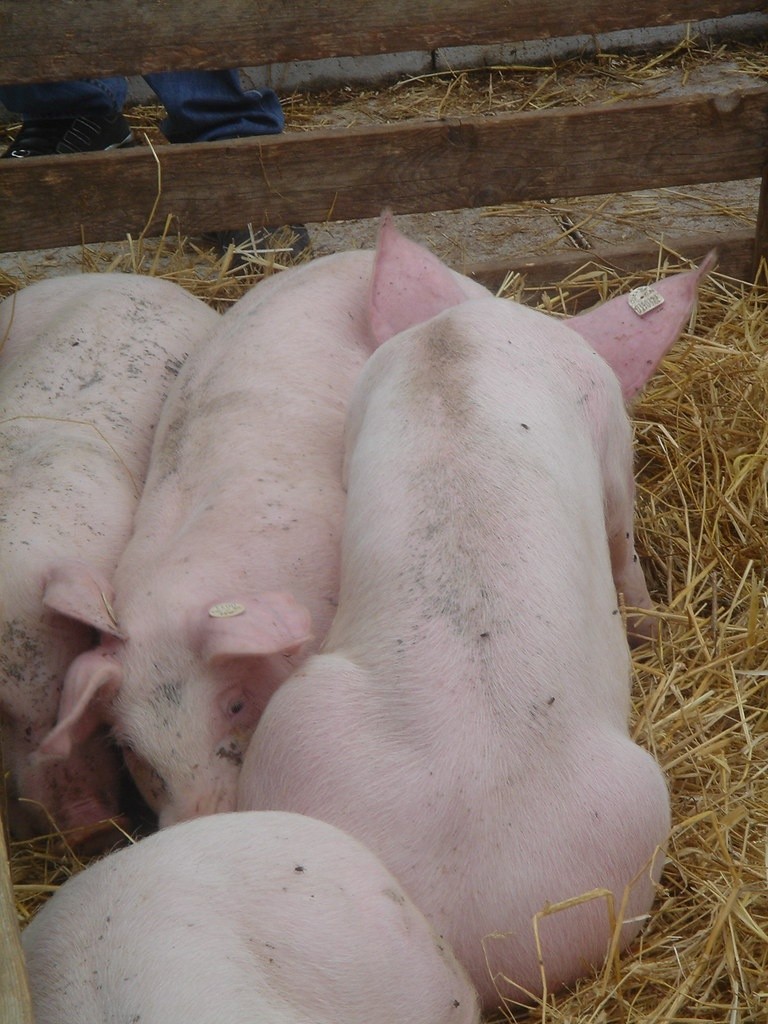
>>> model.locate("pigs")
[0,205,722,1024]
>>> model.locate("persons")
[2,70,313,270]
